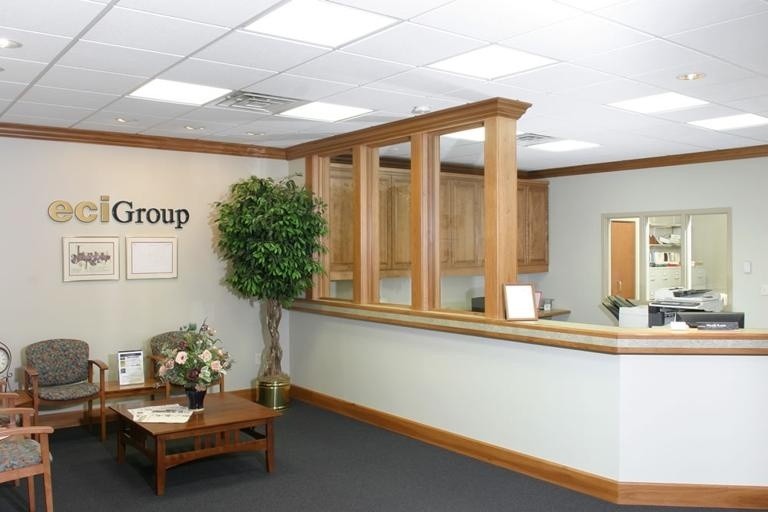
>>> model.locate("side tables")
[93,379,167,441]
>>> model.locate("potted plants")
[215,175,330,414]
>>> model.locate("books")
[658,233,681,244]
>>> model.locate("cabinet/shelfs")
[516,178,550,274]
[648,225,688,304]
[378,170,411,277]
[330,165,354,280]
[441,174,484,275]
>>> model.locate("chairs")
[0,382,19,429]
[148,331,224,400]
[0,408,54,512]
[23,339,108,442]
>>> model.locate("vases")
[186,387,206,411]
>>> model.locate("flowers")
[154,321,236,391]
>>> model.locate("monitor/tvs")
[676,312,746,329]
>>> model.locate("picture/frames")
[62,236,120,282]
[125,236,177,279]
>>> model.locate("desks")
[9,390,34,408]
[109,392,282,495]
